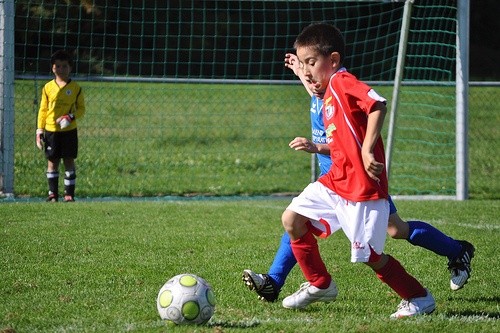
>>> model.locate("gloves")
[56,114,75,130]
[36,129,45,150]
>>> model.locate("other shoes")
[48,195,58,201]
[64,192,74,202]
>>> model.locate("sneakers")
[447,241,475,290]
[242,269,282,303]
[390,289,435,319]
[282,275,338,309]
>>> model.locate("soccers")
[158,274,216,325]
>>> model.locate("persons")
[35,49,85,202]
[282,23,435,319]
[242,53,476,302]
